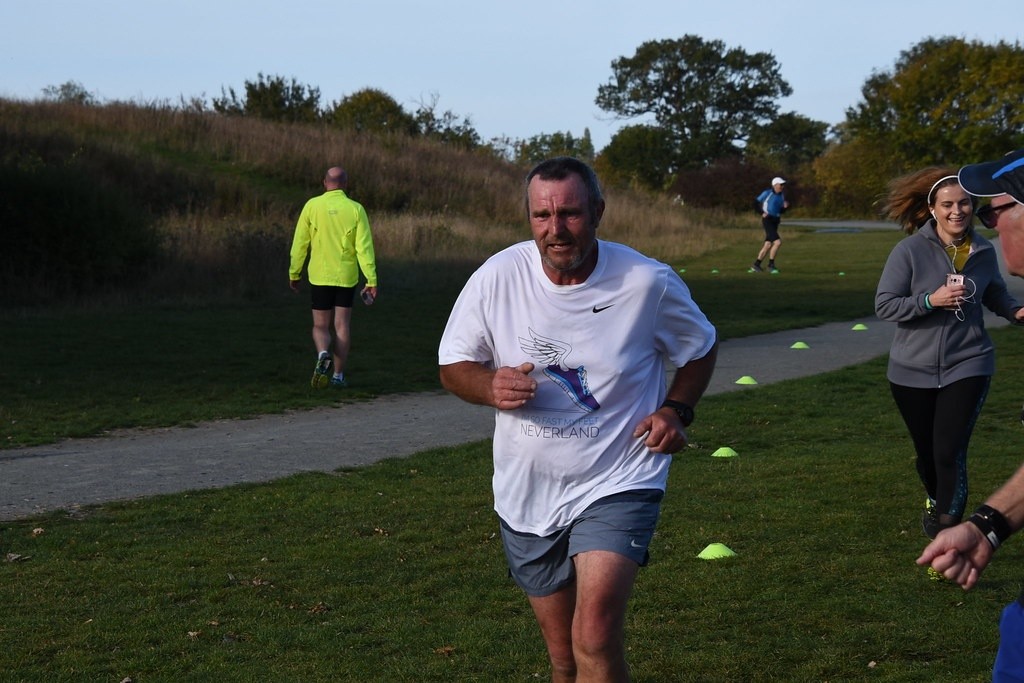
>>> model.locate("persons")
[874,165,1023,547]
[915,142,1024,683]
[289,166,377,389]
[750,176,791,272]
[438,157,721,683]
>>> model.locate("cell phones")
[945,274,964,311]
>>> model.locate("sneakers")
[310,352,332,389]
[333,377,346,388]
[923,495,939,541]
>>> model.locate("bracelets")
[925,293,937,310]
[968,503,1014,553]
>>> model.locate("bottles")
[361,290,373,305]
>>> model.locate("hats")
[772,177,787,185]
[958,150,1024,204]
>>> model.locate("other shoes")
[767,265,779,272]
[751,263,763,275]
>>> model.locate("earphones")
[931,210,936,220]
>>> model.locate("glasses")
[975,202,1017,229]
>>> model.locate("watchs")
[661,401,695,427]
